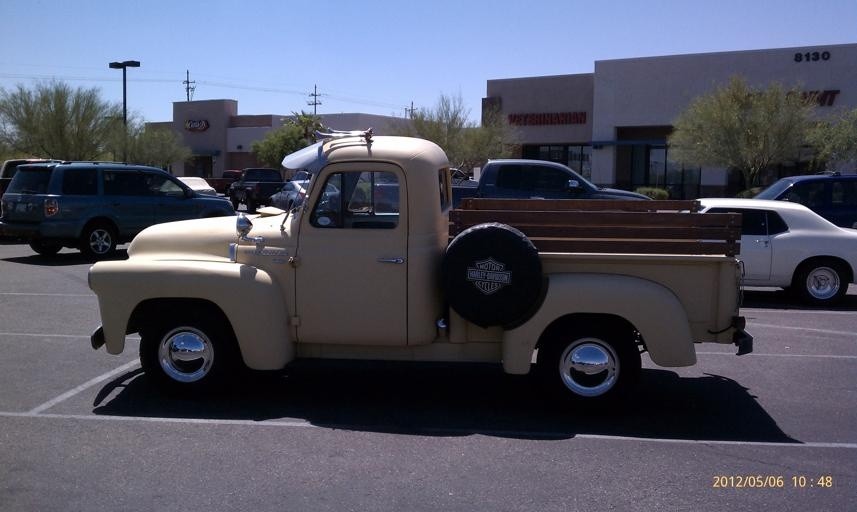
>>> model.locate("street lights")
[109,60,140,163]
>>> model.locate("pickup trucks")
[87,129,753,415]
